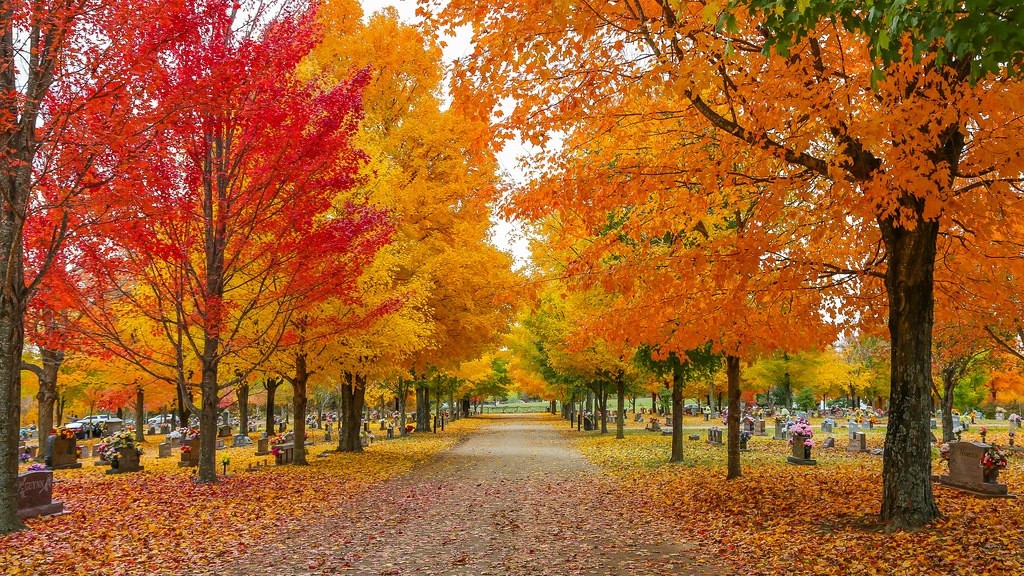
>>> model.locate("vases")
[111,460,119,469]
[806,449,811,460]
[990,467,1000,485]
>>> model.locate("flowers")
[938,442,953,461]
[787,417,815,453]
[739,429,752,443]
[29,462,46,473]
[49,425,75,441]
[100,430,146,461]
[977,442,1009,469]
[568,405,1022,424]
[17,410,450,456]
[181,445,192,454]
